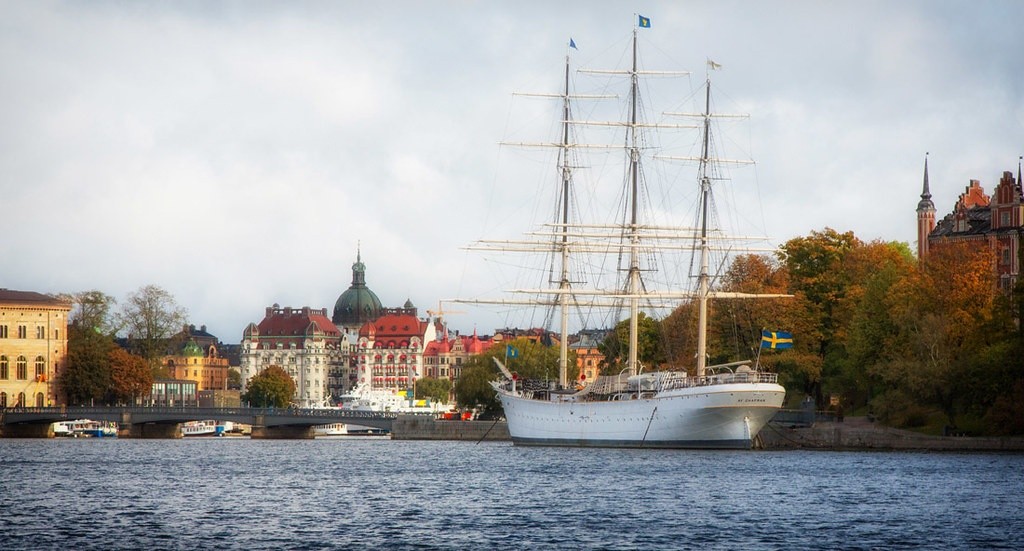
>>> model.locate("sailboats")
[437,11,800,451]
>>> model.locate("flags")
[706,58,721,70]
[639,16,651,28]
[761,331,793,348]
[508,345,518,358]
[569,38,576,49]
